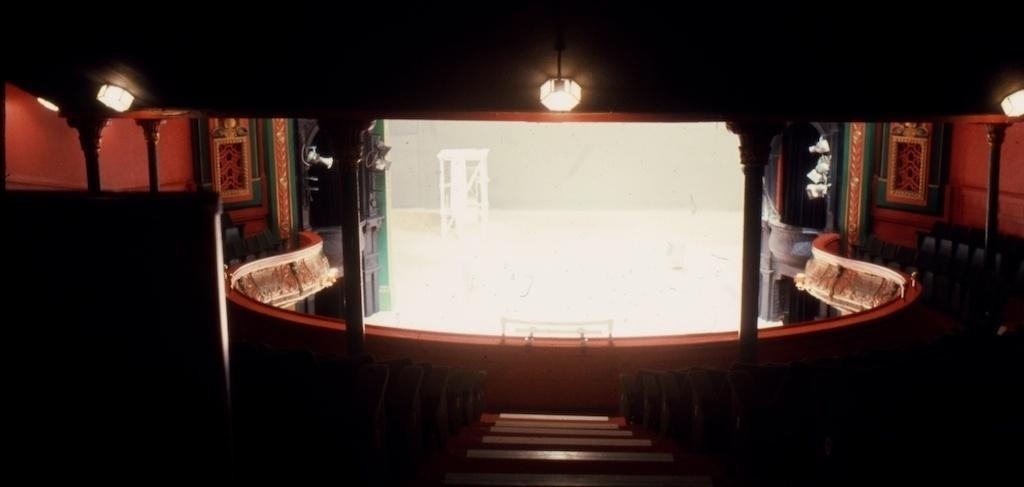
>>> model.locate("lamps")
[536,23,584,112]
[90,80,142,113]
